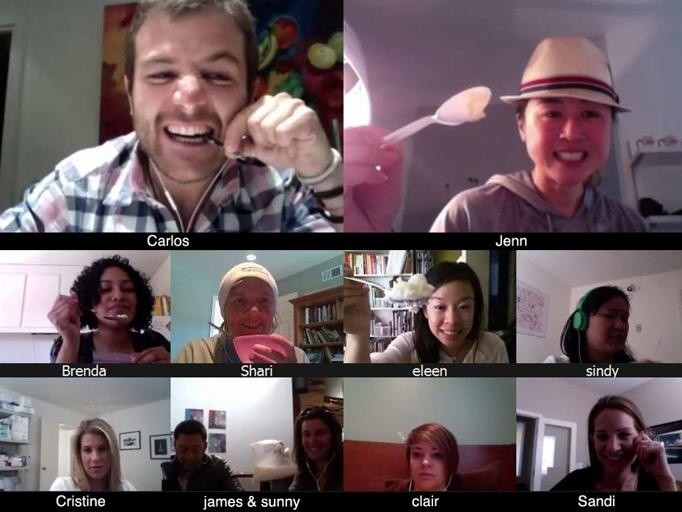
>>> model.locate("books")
[152,295,163,316]
[161,294,170,316]
[343,251,433,352]
[300,299,343,363]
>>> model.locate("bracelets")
[311,184,345,201]
[317,207,345,224]
[312,197,344,210]
[295,145,343,185]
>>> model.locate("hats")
[499,36,631,114]
[217,262,279,317]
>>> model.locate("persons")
[170,418,245,492]
[384,422,470,491]
[0,1,345,235]
[286,407,343,492]
[344,33,650,232]
[548,395,678,493]
[48,417,139,492]
[45,254,171,364]
[540,284,663,364]
[174,261,313,364]
[343,260,511,364]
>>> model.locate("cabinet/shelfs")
[288,283,345,349]
[0,404,35,470]
[622,141,682,232]
[343,250,414,338]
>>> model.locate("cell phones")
[632,439,653,473]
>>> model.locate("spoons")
[381,86,493,151]
[78,304,127,324]
[343,273,435,306]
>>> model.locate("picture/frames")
[119,431,141,450]
[150,434,171,459]
[647,420,682,463]
[516,279,549,338]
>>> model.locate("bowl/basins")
[234,335,283,364]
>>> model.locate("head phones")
[573,288,595,333]
